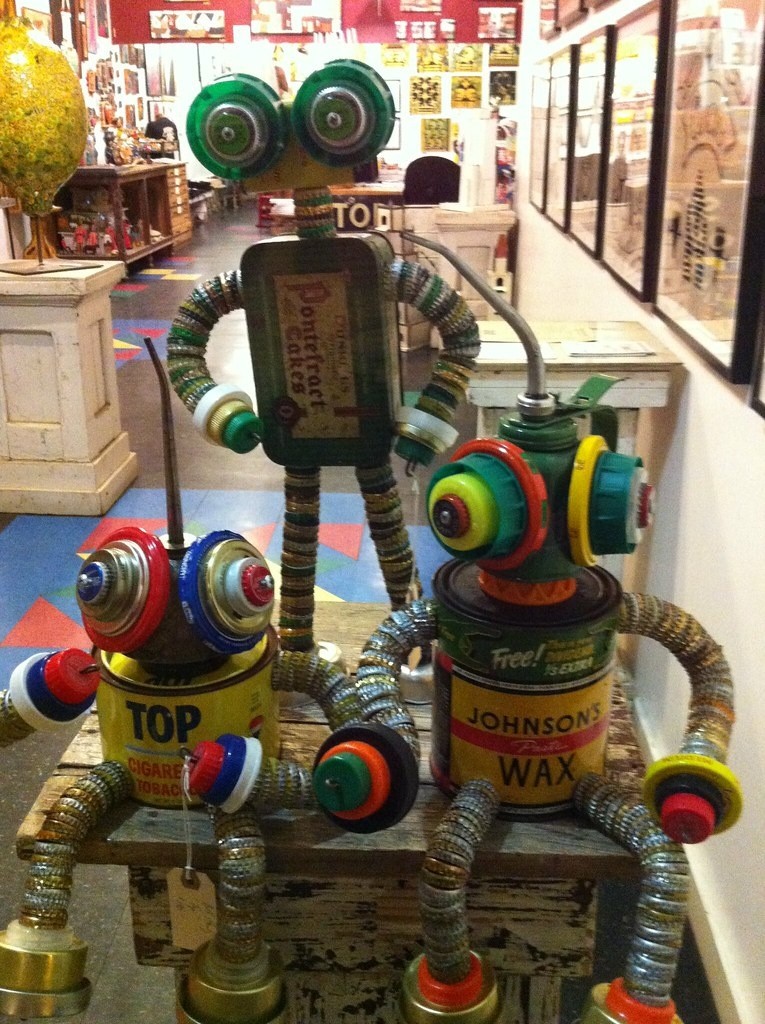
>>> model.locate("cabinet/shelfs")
[56,163,173,275]
[142,159,192,247]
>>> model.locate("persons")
[71,216,141,255]
[145,101,181,161]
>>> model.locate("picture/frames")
[599,1,675,305]
[560,21,614,260]
[527,55,552,216]
[538,41,580,235]
[650,0,765,384]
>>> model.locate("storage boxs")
[69,187,103,214]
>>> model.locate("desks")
[465,313,682,590]
[14,599,647,1024]
